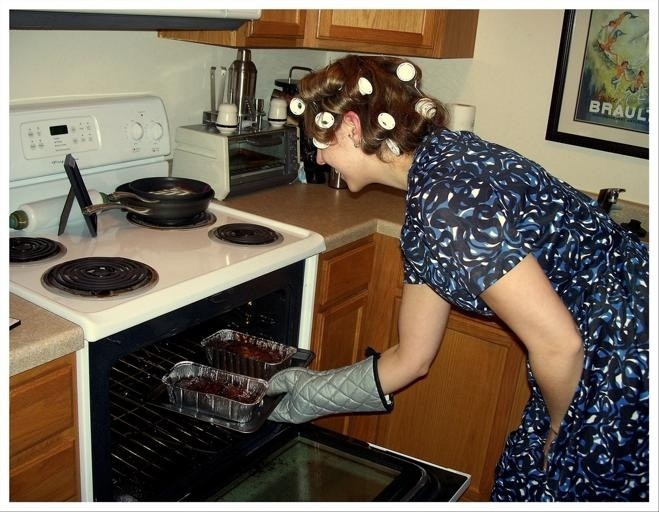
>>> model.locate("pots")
[87,176,216,219]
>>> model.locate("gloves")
[266,355,395,424]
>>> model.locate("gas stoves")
[8,181,325,342]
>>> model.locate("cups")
[269,99,288,128]
[214,104,238,134]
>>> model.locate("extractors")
[10,8,261,30]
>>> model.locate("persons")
[265,50,651,504]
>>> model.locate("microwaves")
[169,118,301,200]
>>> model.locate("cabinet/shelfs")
[304,9,479,58]
[311,233,376,441]
[158,9,304,49]
[10,349,84,502]
[346,232,529,501]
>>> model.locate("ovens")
[76,255,473,502]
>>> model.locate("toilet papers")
[444,103,475,133]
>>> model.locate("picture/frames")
[545,9,649,160]
